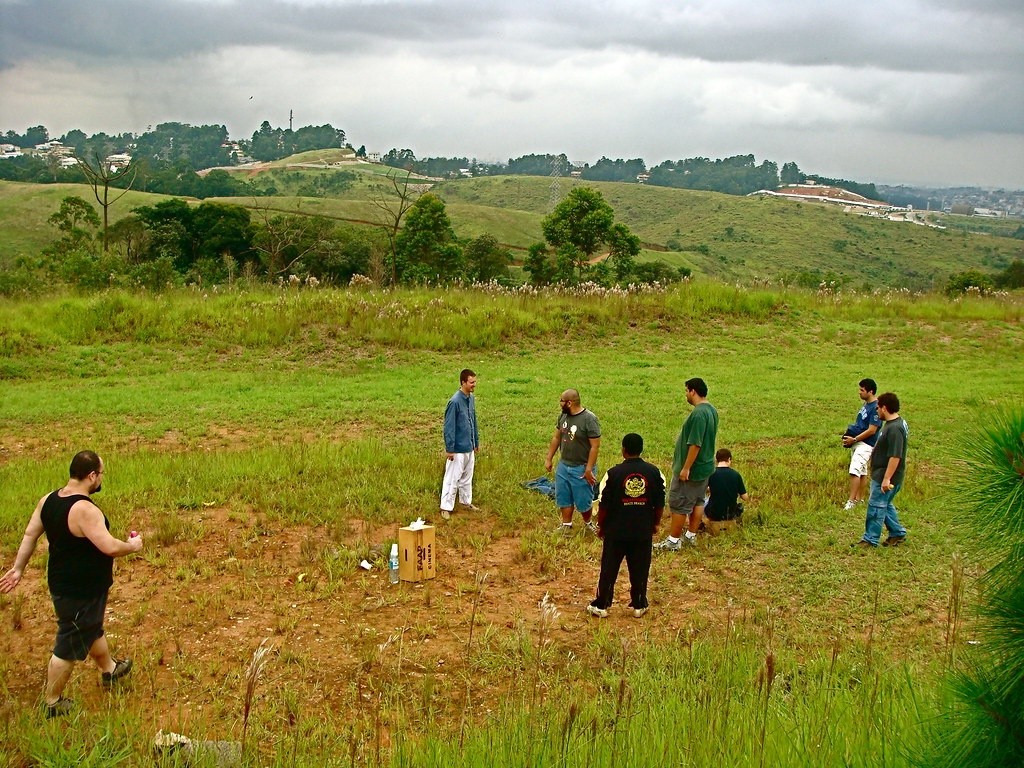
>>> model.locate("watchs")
[853,438,857,443]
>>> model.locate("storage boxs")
[705,519,741,536]
[399,525,436,582]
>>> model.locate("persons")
[544,388,602,536]
[0,449,143,719]
[440,369,482,520]
[704,448,749,521]
[850,392,909,552]
[586,433,667,618]
[652,377,720,550]
[842,378,883,511]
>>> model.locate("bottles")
[388,551,400,584]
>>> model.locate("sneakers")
[654,537,682,551]
[851,539,879,551]
[842,499,856,511]
[881,536,905,548]
[684,531,699,547]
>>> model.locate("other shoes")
[465,502,482,512]
[44,693,80,720]
[438,509,453,520]
[585,603,611,617]
[99,655,134,682]
[631,607,652,618]
